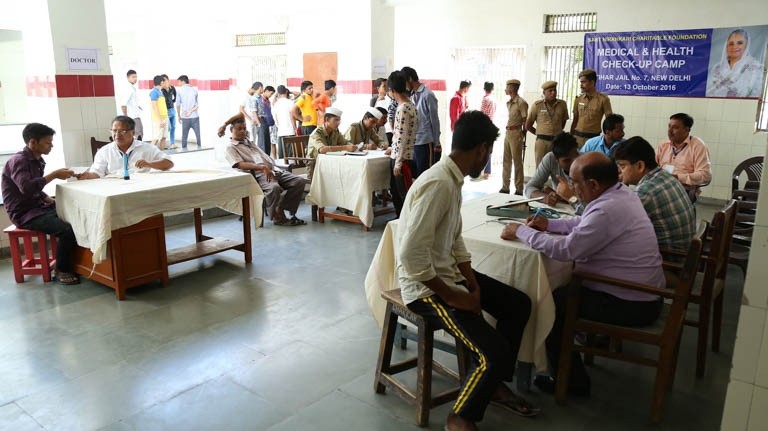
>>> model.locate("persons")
[399,67,443,176]
[72,116,175,181]
[242,80,336,160]
[175,75,202,150]
[306,107,359,215]
[482,81,496,180]
[498,79,528,196]
[387,70,419,220]
[708,29,764,98]
[500,151,667,398]
[397,110,541,431]
[121,70,144,141]
[579,114,628,157]
[525,81,569,169]
[149,74,180,149]
[218,112,308,226]
[570,68,613,150]
[1,123,80,283]
[655,113,712,204]
[525,131,584,205]
[346,78,396,207]
[556,137,695,289]
[450,79,471,131]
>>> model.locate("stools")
[372,287,471,427]
[3,224,56,283]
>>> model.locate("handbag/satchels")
[263,102,274,126]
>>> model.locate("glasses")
[109,129,132,133]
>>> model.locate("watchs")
[569,196,578,209]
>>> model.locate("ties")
[119,151,132,179]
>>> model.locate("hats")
[506,79,521,85]
[579,69,594,78]
[541,81,558,89]
[367,107,383,119]
[326,107,342,116]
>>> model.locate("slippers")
[54,271,80,285]
[490,394,541,417]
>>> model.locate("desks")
[360,190,584,393]
[54,160,264,303]
[305,147,399,233]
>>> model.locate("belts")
[536,135,555,140]
[505,126,522,130]
[573,129,600,138]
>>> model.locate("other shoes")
[513,191,523,195]
[471,176,489,181]
[499,189,510,194]
[291,217,307,225]
[532,374,591,394]
[374,196,382,204]
[377,193,392,201]
[595,332,623,350]
[274,217,297,226]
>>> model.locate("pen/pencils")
[531,207,541,221]
[369,139,374,144]
[347,141,353,145]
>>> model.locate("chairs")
[659,201,738,378]
[551,236,699,427]
[280,136,323,223]
[731,156,767,274]
